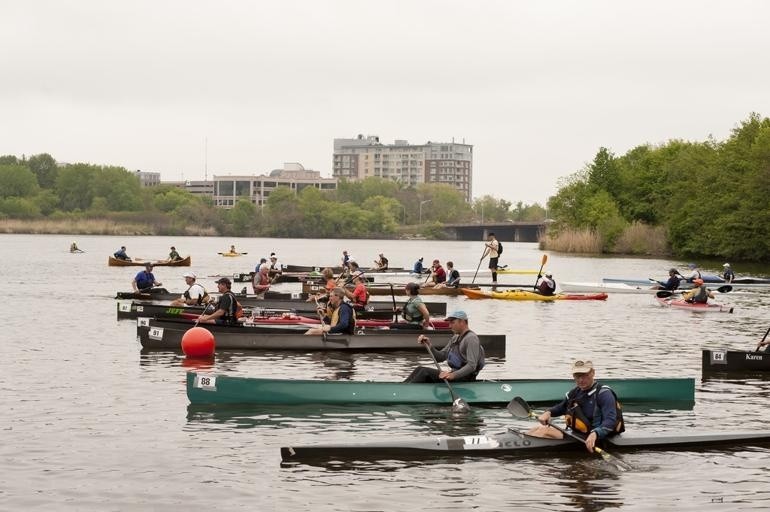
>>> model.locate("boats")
[277,429,770,465]
[460,285,609,305]
[114,264,493,322]
[130,316,509,360]
[108,255,193,270]
[699,346,769,377]
[603,275,770,290]
[182,367,700,408]
[556,278,717,295]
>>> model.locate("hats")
[692,279,703,284]
[722,262,730,267]
[214,278,232,285]
[571,359,593,374]
[444,310,468,322]
[146,262,155,267]
[350,271,365,279]
[183,271,196,278]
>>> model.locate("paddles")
[656,285,732,298]
[507,396,635,470]
[256,273,281,301]
[318,310,327,345]
[422,338,471,410]
[535,255,547,291]
[218,253,248,255]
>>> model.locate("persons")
[303,249,367,335]
[370,251,460,290]
[392,281,430,327]
[647,262,734,304]
[533,271,556,297]
[481,232,499,284]
[524,359,625,454]
[230,245,235,253]
[113,246,132,261]
[170,271,206,308]
[131,262,169,295]
[403,310,485,384]
[198,277,237,326]
[167,247,184,261]
[252,251,282,295]
